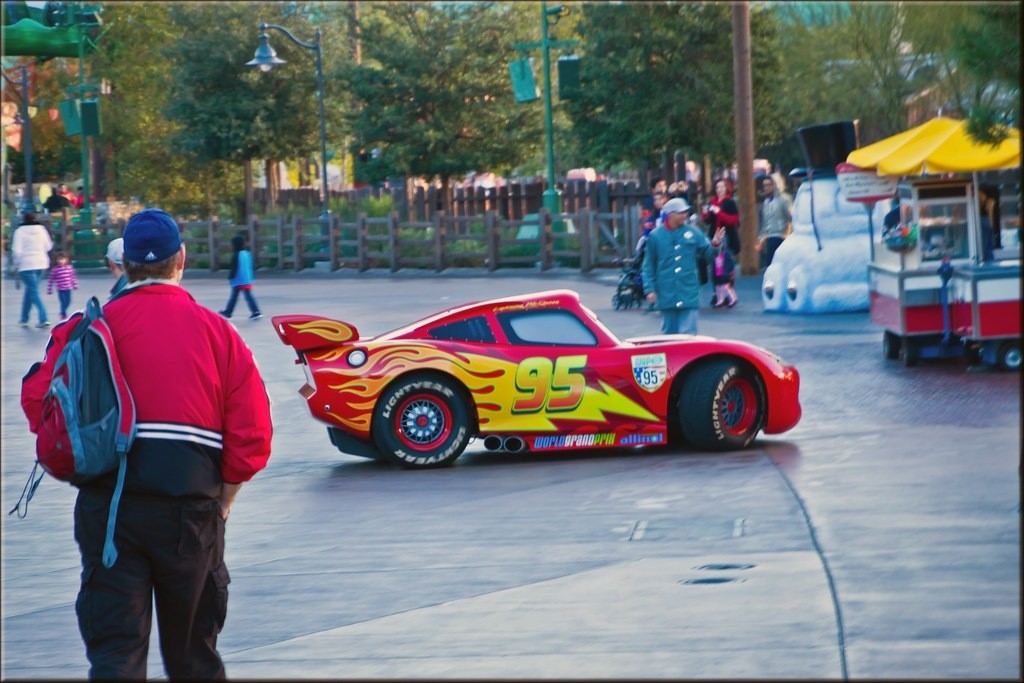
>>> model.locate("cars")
[272,288,803,469]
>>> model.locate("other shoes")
[218,310,231,321]
[36,320,51,327]
[715,296,729,308]
[19,320,28,329]
[727,299,738,307]
[249,313,263,320]
[61,315,67,321]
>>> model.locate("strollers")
[611,234,654,313]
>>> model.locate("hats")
[106,238,126,264]
[122,207,182,263]
[660,197,691,215]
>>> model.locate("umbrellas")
[848,115,1022,178]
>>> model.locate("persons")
[702,178,741,307]
[105,237,129,300]
[11,213,53,328]
[217,236,264,320]
[74,207,273,680]
[47,251,78,320]
[755,176,793,263]
[962,205,995,260]
[644,177,689,238]
[643,197,726,335]
[46,184,94,213]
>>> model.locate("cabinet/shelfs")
[900,196,975,268]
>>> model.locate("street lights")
[245,22,332,253]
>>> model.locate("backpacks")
[8,294,139,484]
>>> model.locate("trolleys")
[847,115,1022,373]
